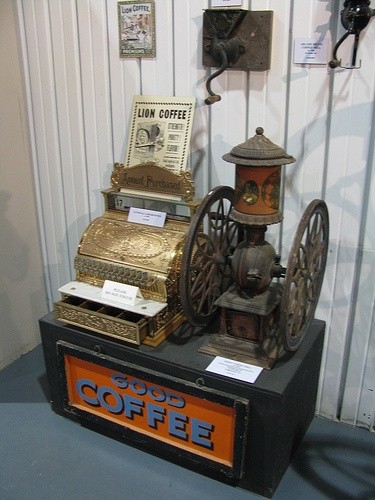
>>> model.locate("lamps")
[328,0,375,70]
[222,127,296,246]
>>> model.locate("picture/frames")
[117,1,156,59]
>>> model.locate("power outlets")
[202,8,273,70]
